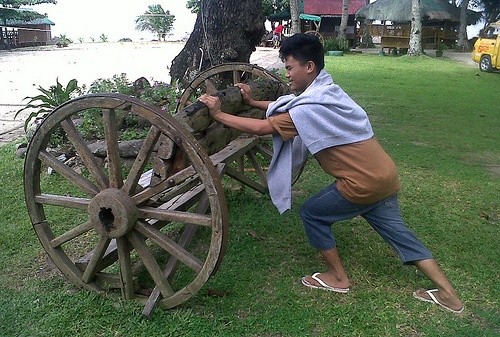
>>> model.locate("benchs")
[381,37,411,54]
[121,78,268,190]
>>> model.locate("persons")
[195,33,465,314]
[272,24,288,48]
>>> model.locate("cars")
[472,20,500,72]
[150,25,287,49]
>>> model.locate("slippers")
[301,272,350,294]
[412,287,464,315]
